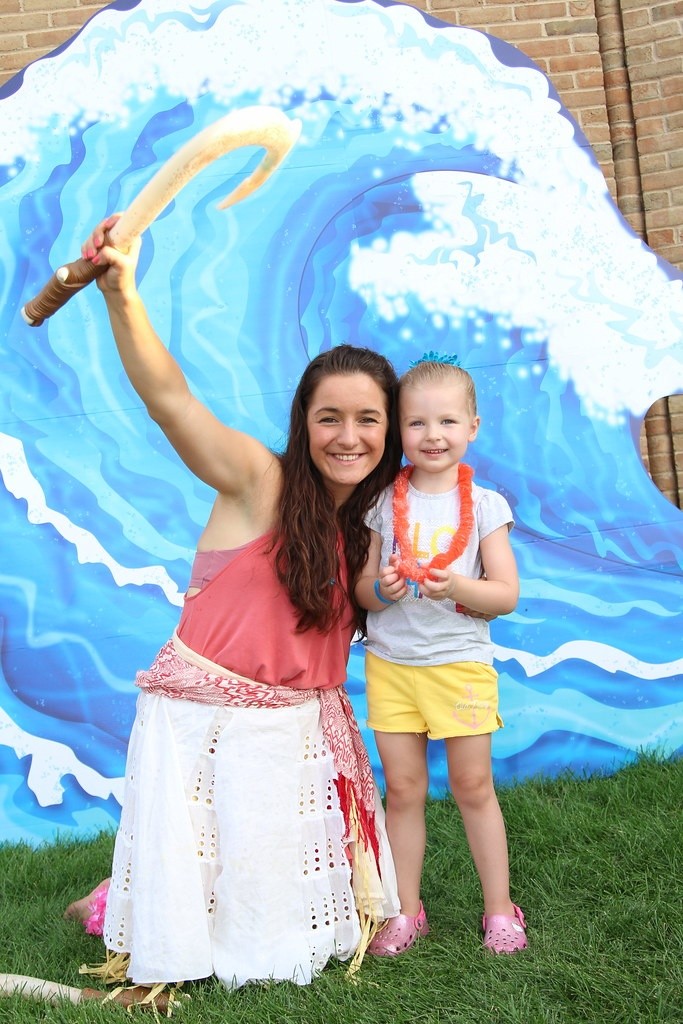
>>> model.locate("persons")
[355,362,530,958]
[62,215,407,989]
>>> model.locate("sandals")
[482,902,529,956]
[368,899,428,959]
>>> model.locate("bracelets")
[373,580,398,605]
